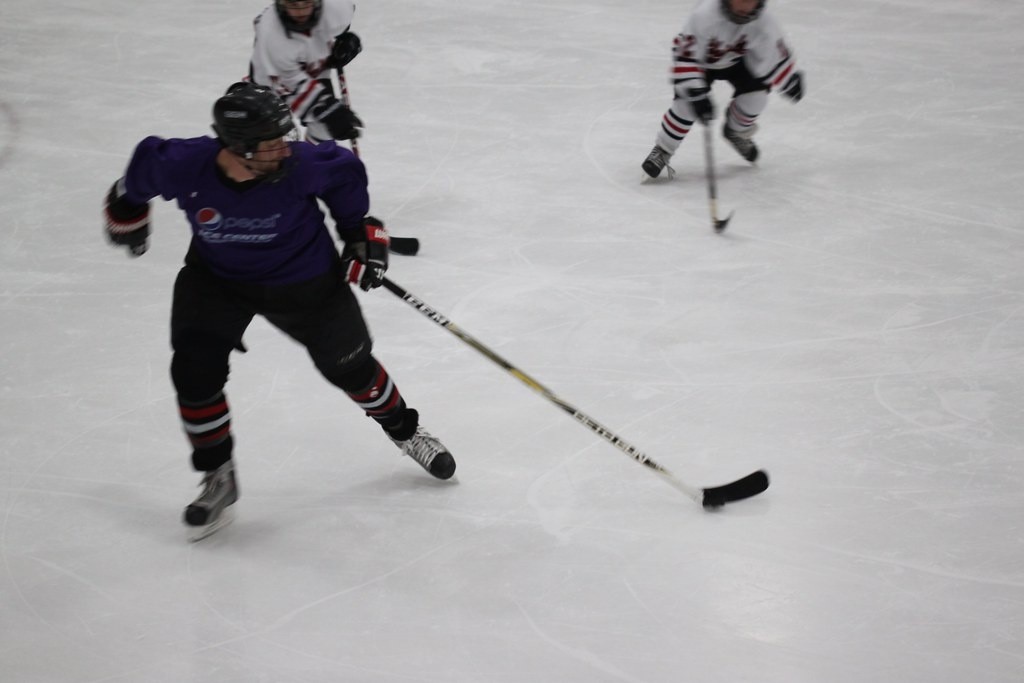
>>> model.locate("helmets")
[211,82,295,161]
[721,0,766,25]
[276,0,322,38]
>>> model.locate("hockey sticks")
[336,64,419,258]
[375,264,770,506]
[703,120,735,232]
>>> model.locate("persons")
[103,80,457,526]
[641,0,808,178]
[246,0,362,147]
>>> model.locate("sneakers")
[642,145,675,182]
[724,124,758,162]
[184,457,237,542]
[387,430,455,483]
